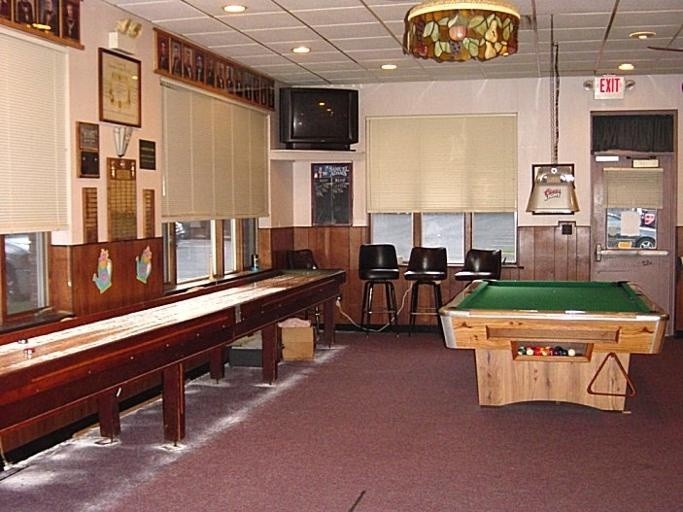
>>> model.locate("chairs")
[286,243,503,337]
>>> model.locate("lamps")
[526,13,581,216]
[403,0,519,63]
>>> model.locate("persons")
[158,41,274,109]
[63,2,78,39]
[639,210,656,230]
[17,0,34,27]
[41,0,57,35]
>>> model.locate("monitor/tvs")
[278,87,359,151]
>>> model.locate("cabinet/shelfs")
[0,266,350,456]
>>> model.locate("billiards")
[517,346,575,356]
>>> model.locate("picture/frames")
[149,26,274,115]
[0,2,88,51]
[532,163,575,216]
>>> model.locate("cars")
[606,212,656,248]
[5,237,29,301]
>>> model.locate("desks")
[438,278,670,413]
